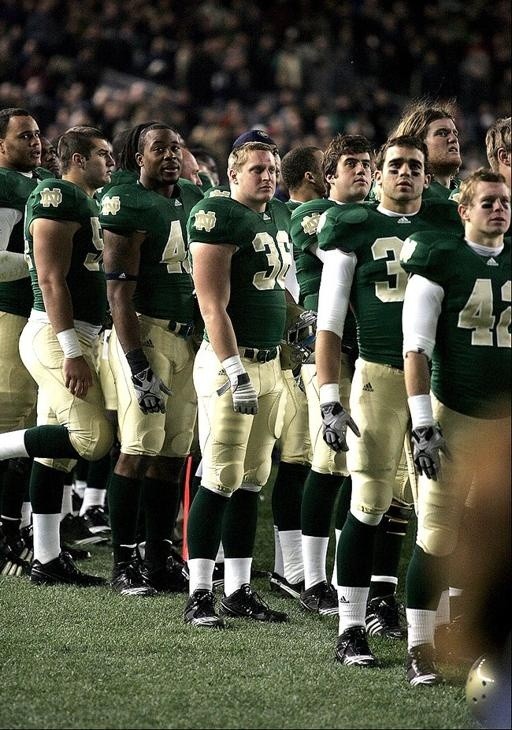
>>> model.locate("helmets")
[465,649,511,730]
[280,301,317,370]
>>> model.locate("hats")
[232,130,276,149]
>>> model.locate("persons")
[4,0,512,642]
[314,138,465,665]
[402,171,512,685]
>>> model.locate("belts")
[169,321,197,338]
[244,346,280,364]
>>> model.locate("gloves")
[411,425,453,482]
[230,373,258,415]
[320,402,360,453]
[131,362,174,415]
